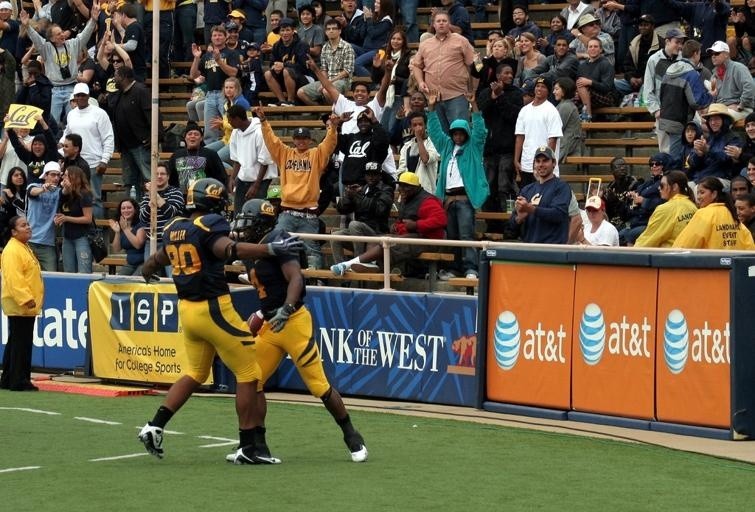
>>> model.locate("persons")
[226,198,369,465]
[137,178,308,466]
[1,0,755,289]
[0,216,45,390]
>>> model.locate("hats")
[39,161,61,179]
[247,42,260,50]
[272,18,295,34]
[392,172,420,187]
[73,83,90,95]
[184,121,202,136]
[578,13,730,52]
[701,103,735,124]
[534,77,552,92]
[225,10,246,31]
[584,196,605,210]
[535,146,556,159]
[69,93,75,101]
[292,127,311,138]
[362,162,382,173]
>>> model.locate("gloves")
[140,266,159,284]
[267,304,296,333]
[268,235,306,257]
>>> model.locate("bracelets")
[282,302,297,315]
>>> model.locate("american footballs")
[247,310,264,337]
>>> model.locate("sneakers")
[138,421,166,460]
[235,448,281,465]
[330,260,381,275]
[226,446,272,461]
[343,431,369,462]
[281,101,295,106]
[268,101,283,107]
[580,113,592,122]
[437,270,478,280]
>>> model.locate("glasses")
[327,27,339,31]
[48,173,60,177]
[111,59,123,63]
[649,163,665,189]
[709,52,721,56]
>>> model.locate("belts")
[445,196,467,200]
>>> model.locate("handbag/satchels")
[161,122,186,152]
[88,229,108,264]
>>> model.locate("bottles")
[634,98,640,108]
[582,105,588,123]
[129,185,137,199]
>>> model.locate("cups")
[308,255,318,270]
[506,200,514,214]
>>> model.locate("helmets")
[231,199,278,241]
[186,178,232,214]
